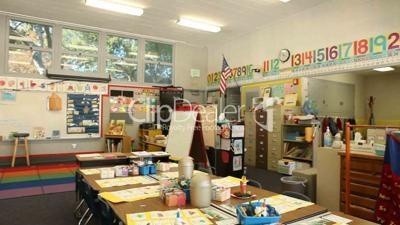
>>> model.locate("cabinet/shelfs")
[281,122,321,167]
[338,151,385,223]
[244,106,302,176]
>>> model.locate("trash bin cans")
[280,176,308,196]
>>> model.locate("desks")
[78,162,382,225]
[74,151,171,168]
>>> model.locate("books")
[283,143,305,158]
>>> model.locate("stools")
[72,173,92,218]
[11,133,31,167]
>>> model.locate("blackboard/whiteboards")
[189,112,207,163]
[165,111,198,161]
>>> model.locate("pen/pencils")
[241,198,276,216]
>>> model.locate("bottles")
[178,158,194,180]
[190,174,212,208]
[323,126,333,147]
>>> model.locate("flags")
[217,57,233,99]
